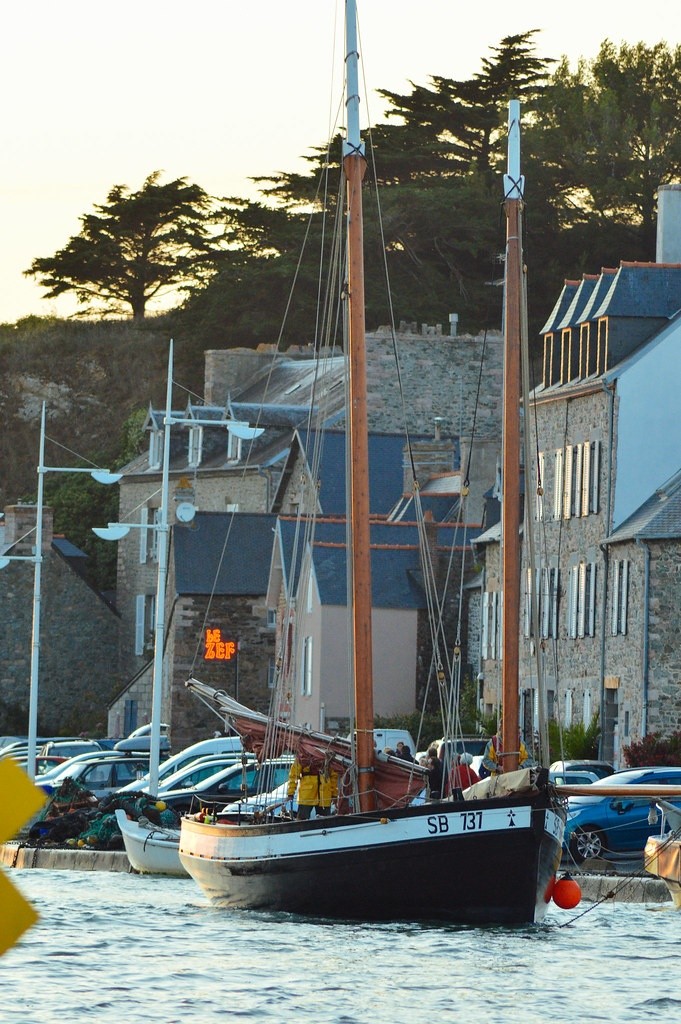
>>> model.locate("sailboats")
[177,0,583,925]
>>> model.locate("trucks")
[346,729,417,758]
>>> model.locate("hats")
[460,753,473,764]
[384,747,391,753]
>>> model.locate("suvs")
[40,740,103,773]
[157,759,294,813]
[563,766,681,862]
[416,734,491,778]
[35,723,173,801]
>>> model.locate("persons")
[286,758,338,820]
[383,725,534,806]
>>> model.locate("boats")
[115,808,189,874]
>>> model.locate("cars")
[550,771,599,784]
[141,760,254,795]
[550,760,616,780]
[35,750,149,782]
[114,736,243,794]
[12,756,69,776]
[161,753,257,784]
